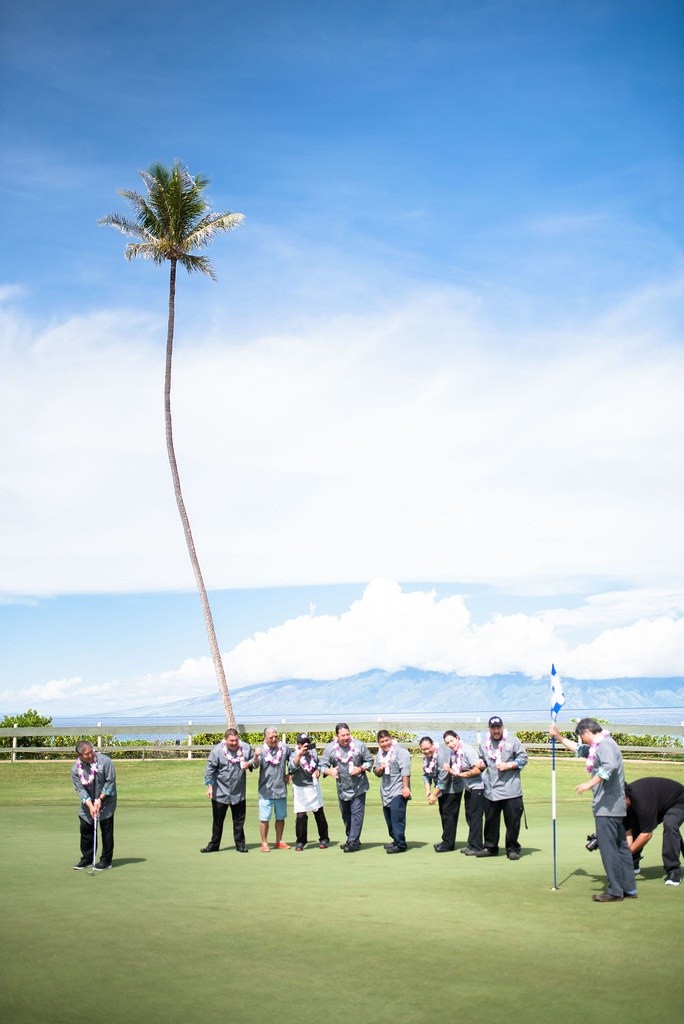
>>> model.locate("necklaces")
[334,735,355,764]
[295,745,316,773]
[265,741,283,765]
[76,750,97,786]
[378,739,395,765]
[423,741,439,774]
[451,739,464,777]
[486,728,507,760]
[222,738,244,764]
[586,729,610,772]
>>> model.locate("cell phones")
[305,743,316,750]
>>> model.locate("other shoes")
[664,872,681,885]
[632,860,641,874]
[464,849,476,855]
[260,844,270,852]
[622,890,638,899]
[319,839,329,848]
[92,860,113,871]
[476,848,499,857]
[339,840,455,855]
[201,845,219,853]
[508,851,519,860]
[275,841,291,849]
[460,846,472,853]
[73,858,93,869]
[592,892,624,902]
[236,845,248,852]
[295,842,307,851]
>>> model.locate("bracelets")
[508,762,512,770]
[98,797,103,801]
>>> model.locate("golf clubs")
[88,812,99,874]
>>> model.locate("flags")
[551,666,566,723]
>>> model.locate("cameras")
[586,833,598,852]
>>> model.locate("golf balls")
[552,888,556,891]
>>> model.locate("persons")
[288,732,330,851]
[71,741,117,871]
[420,736,464,852]
[476,716,528,860]
[200,728,258,853]
[317,723,374,853]
[622,777,684,886]
[252,726,293,852]
[373,730,412,854]
[549,718,637,902]
[443,730,484,856]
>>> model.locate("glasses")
[298,740,311,745]
[420,745,432,752]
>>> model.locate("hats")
[489,716,502,727]
[296,733,310,743]
